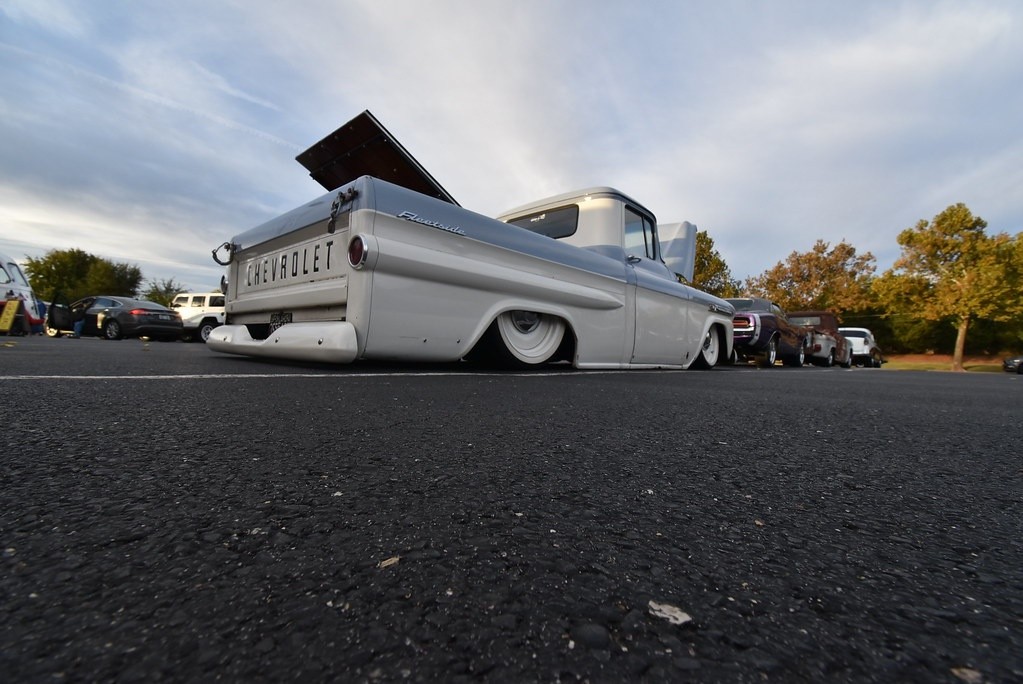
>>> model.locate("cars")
[784,309,853,369]
[1002,355,1023,375]
[722,297,809,369]
[47,291,185,342]
[837,327,882,368]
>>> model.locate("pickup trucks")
[206,107,735,374]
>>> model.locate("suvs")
[169,292,227,344]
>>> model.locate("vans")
[0,254,64,338]
